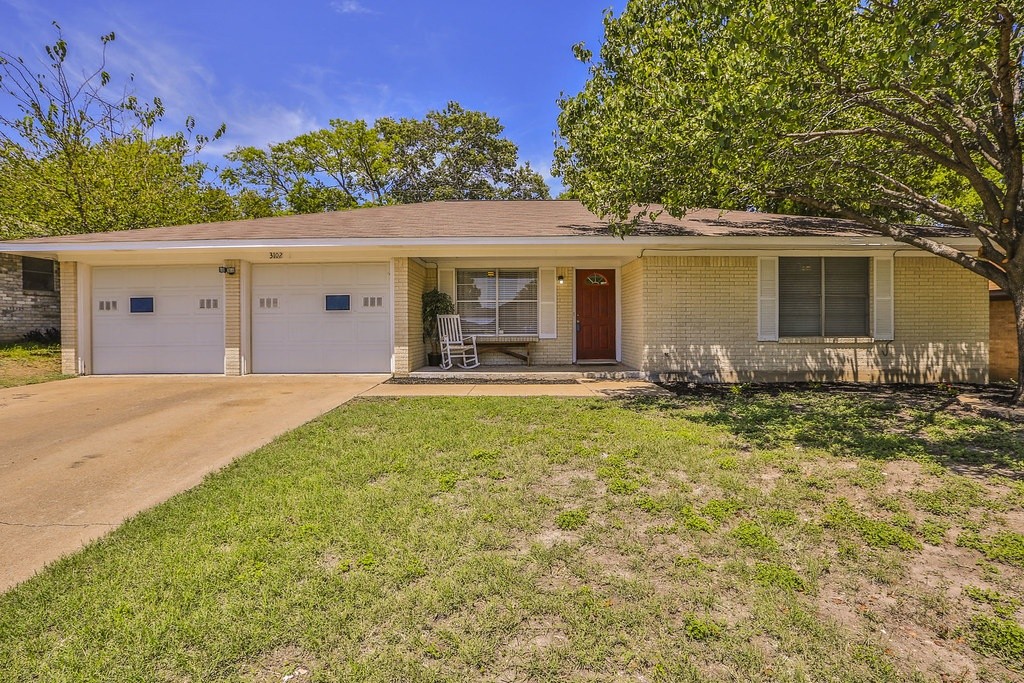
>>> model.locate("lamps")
[227,267,236,274]
[558,276,563,284]
[219,267,226,273]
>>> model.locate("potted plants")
[422,289,455,367]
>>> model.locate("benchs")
[463,341,537,367]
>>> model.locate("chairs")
[436,313,481,369]
